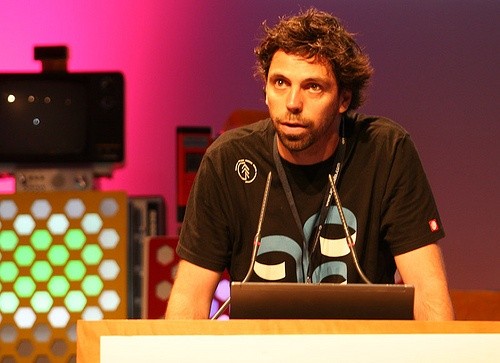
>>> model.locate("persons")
[164,8,457,321]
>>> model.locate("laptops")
[229,282,415,320]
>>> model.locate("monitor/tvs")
[0,72,127,168]
